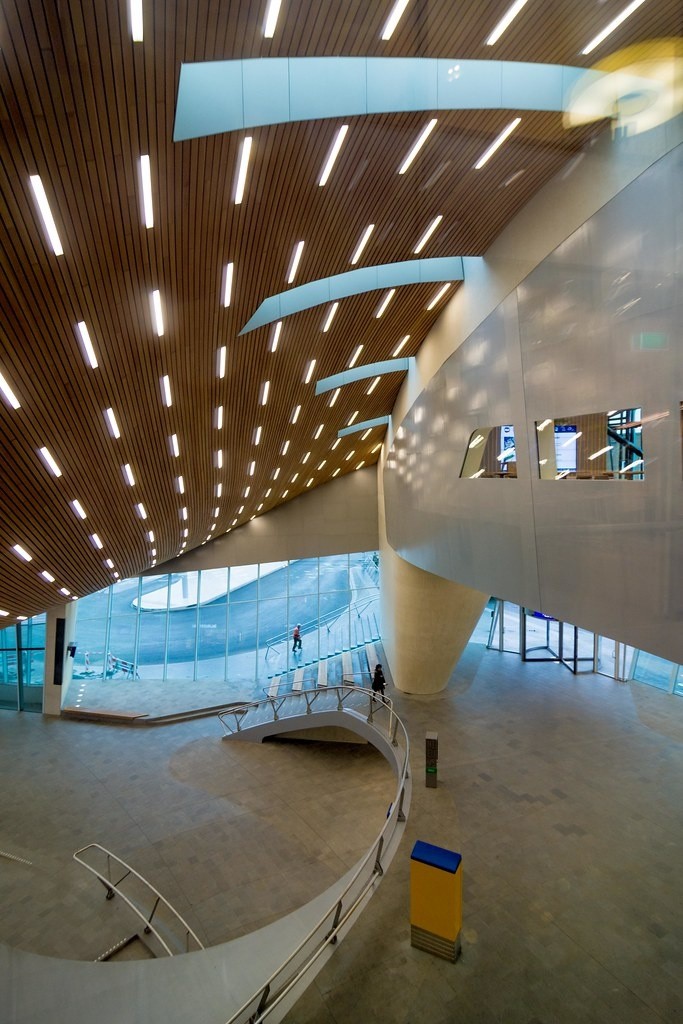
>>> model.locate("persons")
[372,552,379,573]
[291,623,303,652]
[371,664,389,706]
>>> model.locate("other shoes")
[293,650,297,652]
[373,699,377,702]
[298,647,302,649]
[383,701,386,703]
